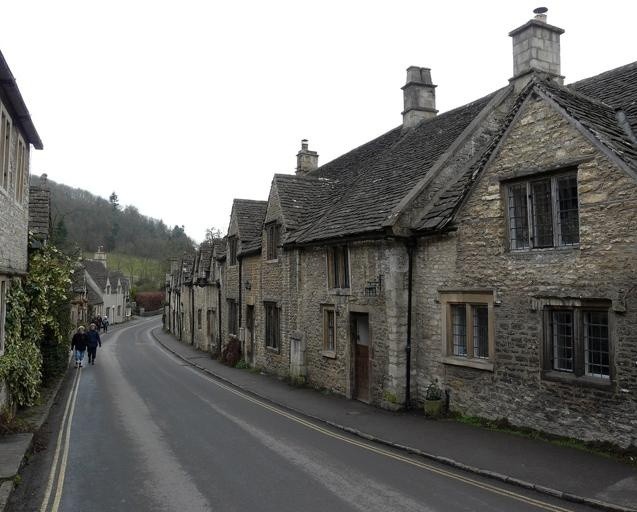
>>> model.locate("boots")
[74,362,83,368]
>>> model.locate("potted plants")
[424,380,443,419]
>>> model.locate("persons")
[161,310,166,328]
[85,322,101,365]
[102,315,108,334]
[70,325,88,369]
[96,313,102,333]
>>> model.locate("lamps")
[244,279,251,290]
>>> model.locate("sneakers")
[89,358,94,365]
